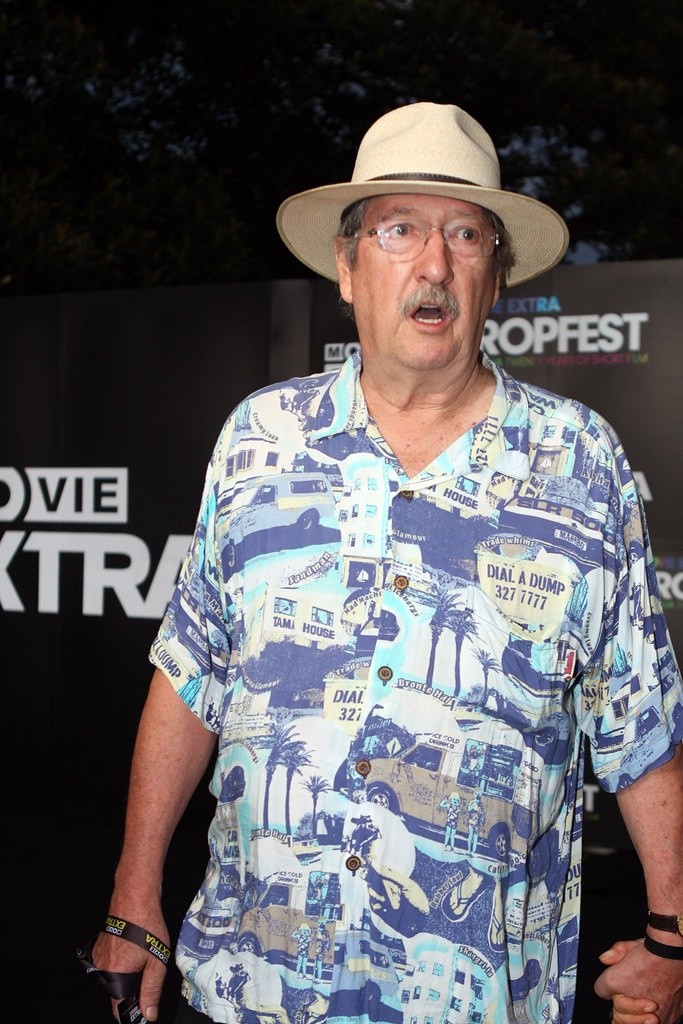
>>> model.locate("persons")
[68,103,683,1024]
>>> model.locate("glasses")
[342,217,513,257]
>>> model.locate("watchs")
[647,910,683,936]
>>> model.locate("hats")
[276,102,570,289]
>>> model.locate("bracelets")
[644,935,683,960]
